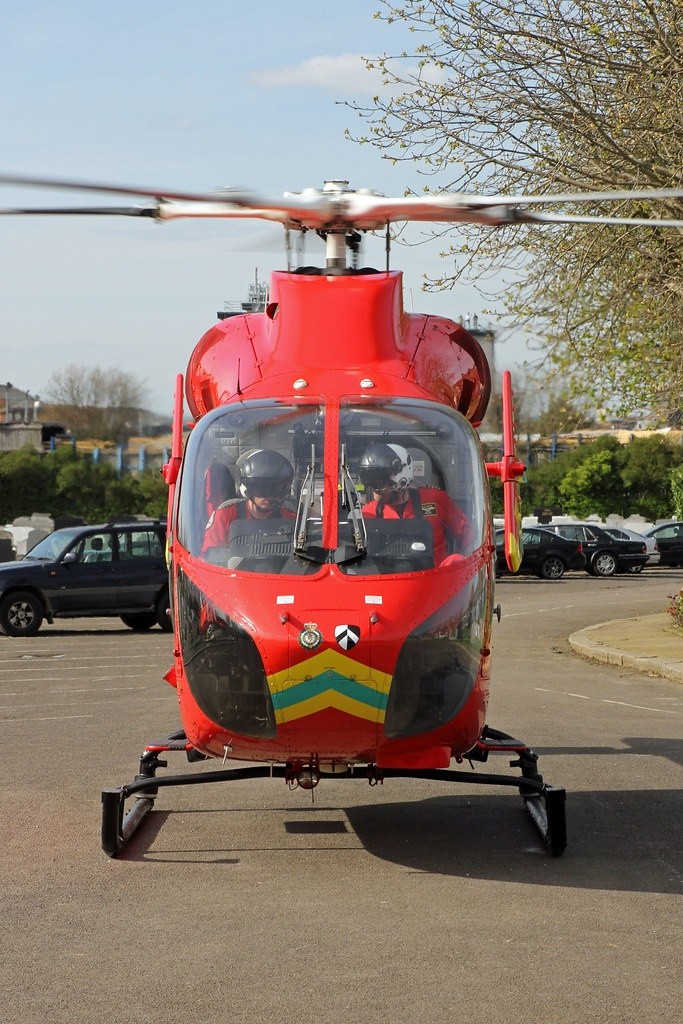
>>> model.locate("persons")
[359,443,476,637]
[196,450,298,633]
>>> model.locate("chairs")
[83,539,123,561]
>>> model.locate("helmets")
[359,443,413,494]
[234,449,294,500]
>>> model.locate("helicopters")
[1,176,683,858]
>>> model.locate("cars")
[491,528,588,580]
[536,523,650,576]
[638,521,682,571]
[601,527,661,571]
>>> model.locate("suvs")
[0,522,173,636]
